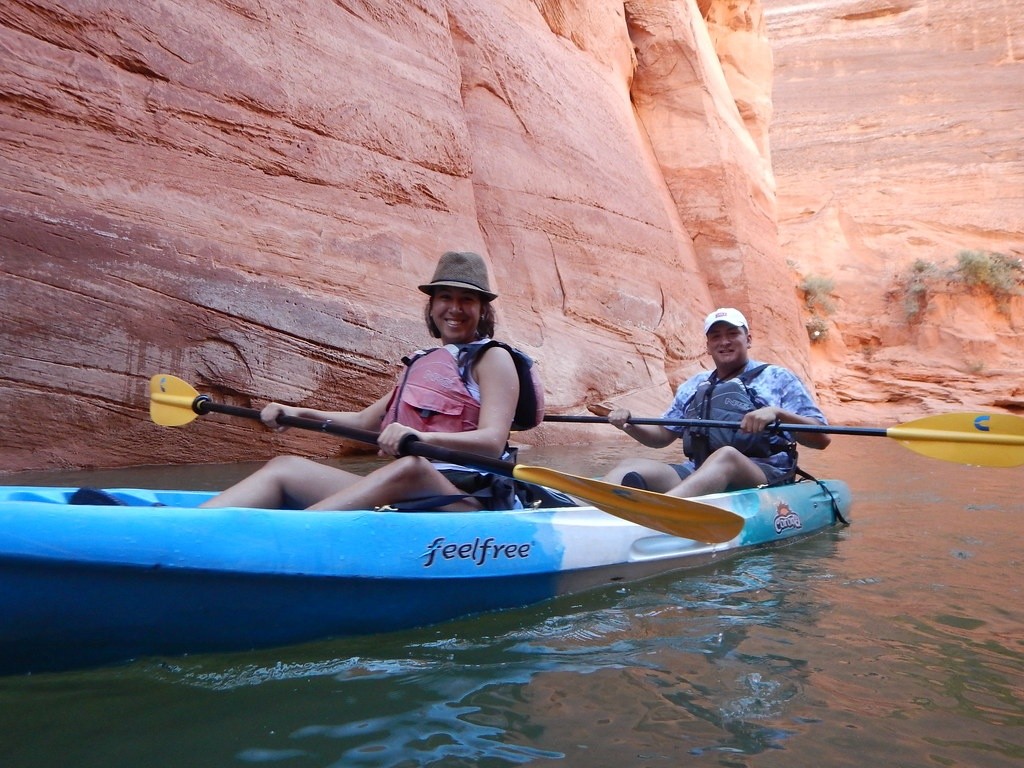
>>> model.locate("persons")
[67,251,520,511]
[519,308,831,508]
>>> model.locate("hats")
[417,250,499,303]
[704,307,750,336]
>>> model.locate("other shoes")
[621,472,647,489]
[68,488,126,506]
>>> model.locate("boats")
[1,476,853,680]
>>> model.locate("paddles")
[147,372,746,547]
[535,410,1024,470]
